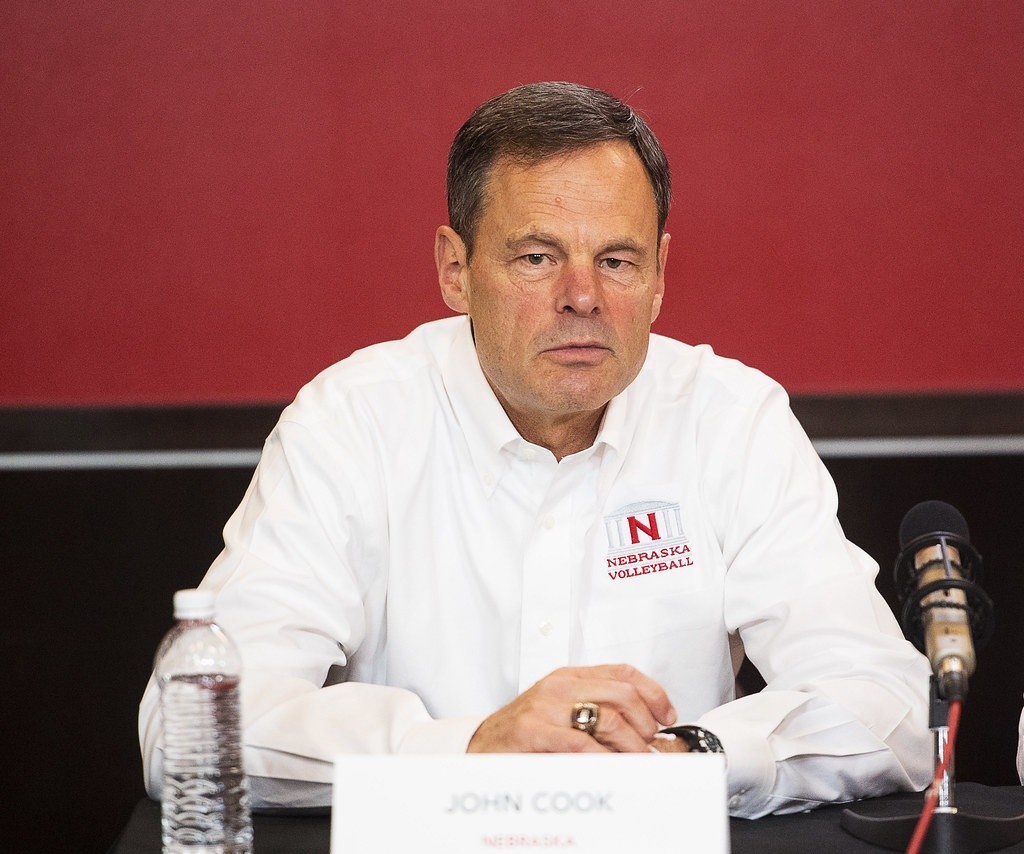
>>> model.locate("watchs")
[659,726,724,753]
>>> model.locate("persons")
[137,83,950,822]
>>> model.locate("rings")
[571,700,600,736]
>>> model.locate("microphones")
[894,501,996,700]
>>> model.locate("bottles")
[153,587,254,854]
